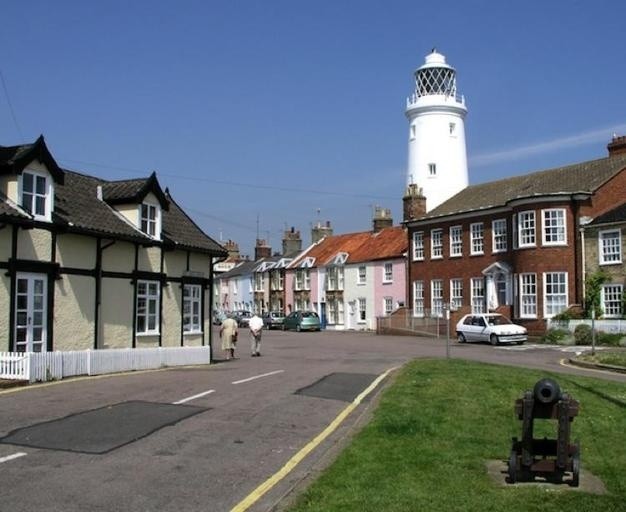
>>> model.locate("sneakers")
[252,352,260,356]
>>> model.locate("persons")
[218,313,239,360]
[248,310,265,357]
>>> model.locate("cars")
[281,310,320,332]
[214,308,251,327]
[454,313,528,346]
[262,311,287,330]
